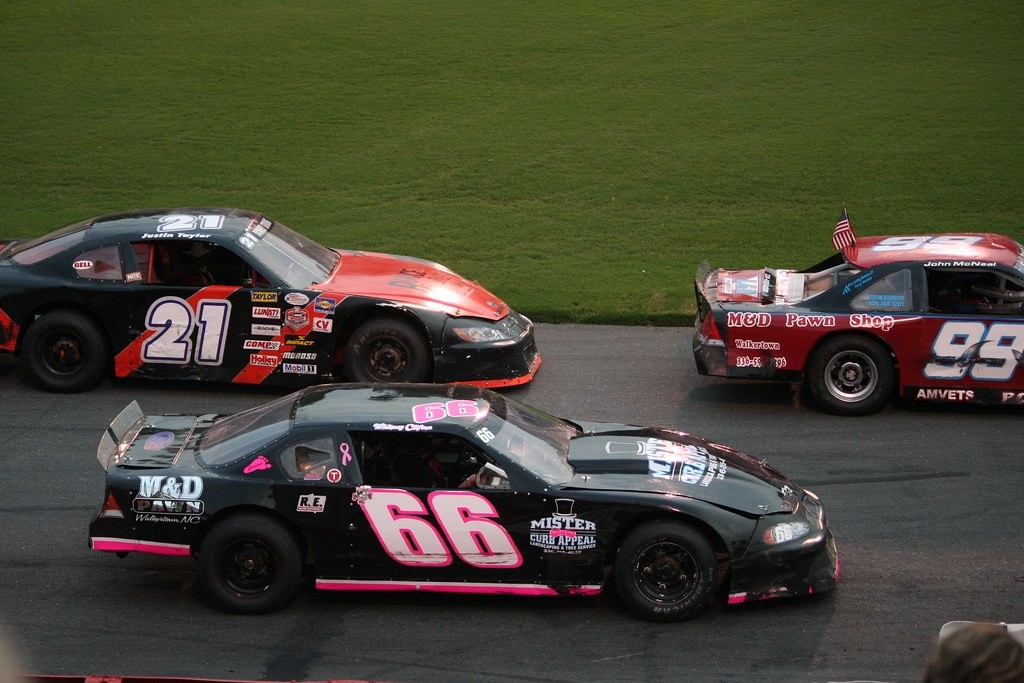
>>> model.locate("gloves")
[441,467,469,488]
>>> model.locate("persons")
[178,240,234,284]
[393,434,485,487]
[934,273,1024,314]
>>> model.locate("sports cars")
[694,234,1024,416]
[85,382,841,619]
[0,207,542,393]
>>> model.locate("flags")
[832,209,856,251]
